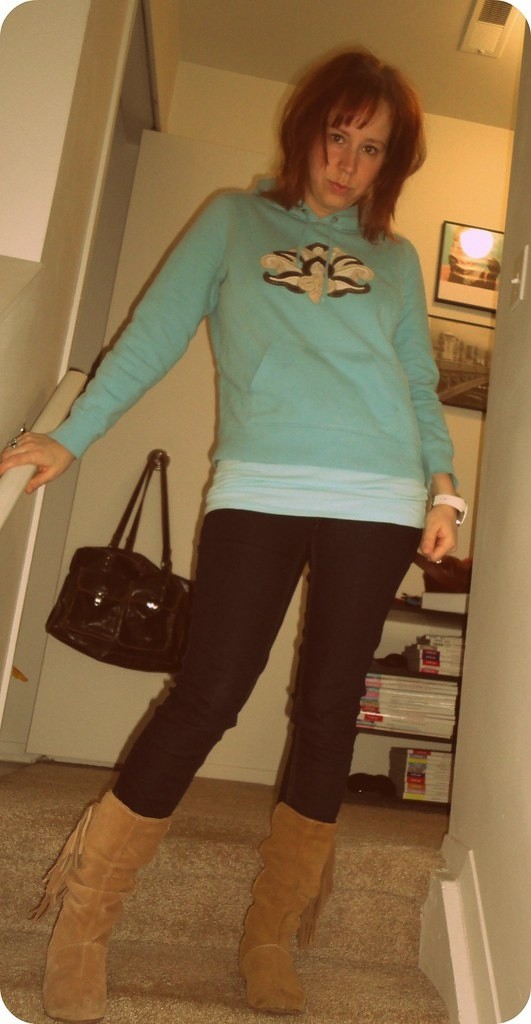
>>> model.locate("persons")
[0,52,469,1024]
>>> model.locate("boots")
[28,787,172,1024]
[237,801,336,1017]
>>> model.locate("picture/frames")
[435,220,505,315]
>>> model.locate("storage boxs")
[421,591,470,614]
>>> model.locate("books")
[402,634,463,676]
[422,592,469,615]
[355,673,458,739]
[389,747,451,802]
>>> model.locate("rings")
[436,559,442,564]
[9,439,17,448]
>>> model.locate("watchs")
[428,494,469,528]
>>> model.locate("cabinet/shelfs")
[342,599,468,814]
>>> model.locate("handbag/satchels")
[45,454,195,674]
